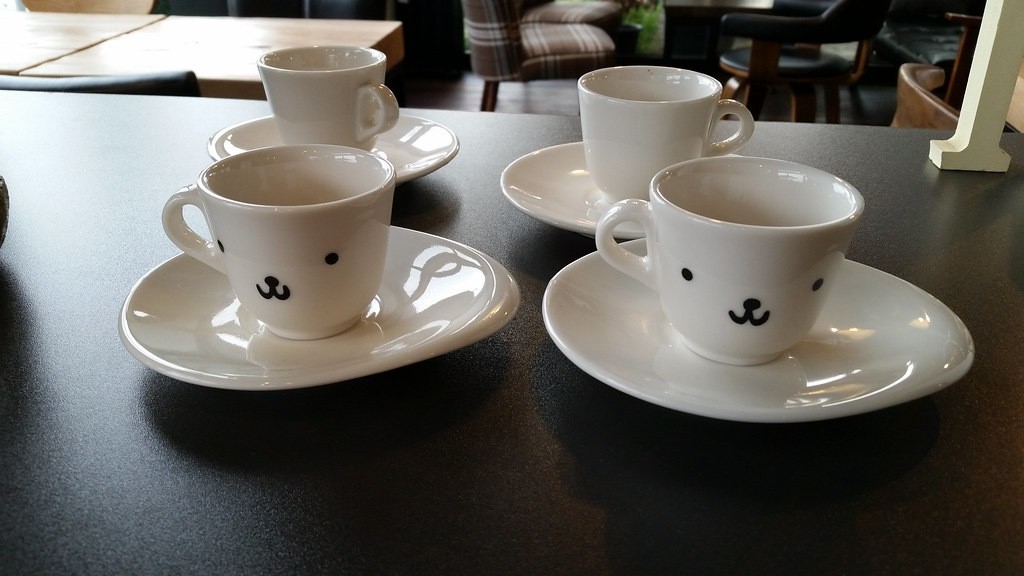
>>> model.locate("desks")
[0,10,167,77]
[627,0,775,74]
[18,16,405,104]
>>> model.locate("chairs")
[716,0,893,124]
[888,62,962,129]
[868,0,987,112]
[461,0,616,111]
[514,0,625,32]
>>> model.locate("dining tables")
[0,89,1024,576]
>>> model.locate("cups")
[256,45,399,151]
[162,145,396,340]
[595,156,865,366]
[577,66,755,205]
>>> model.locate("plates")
[543,237,973,423]
[500,141,740,238]
[207,116,460,184]
[118,224,520,389]
